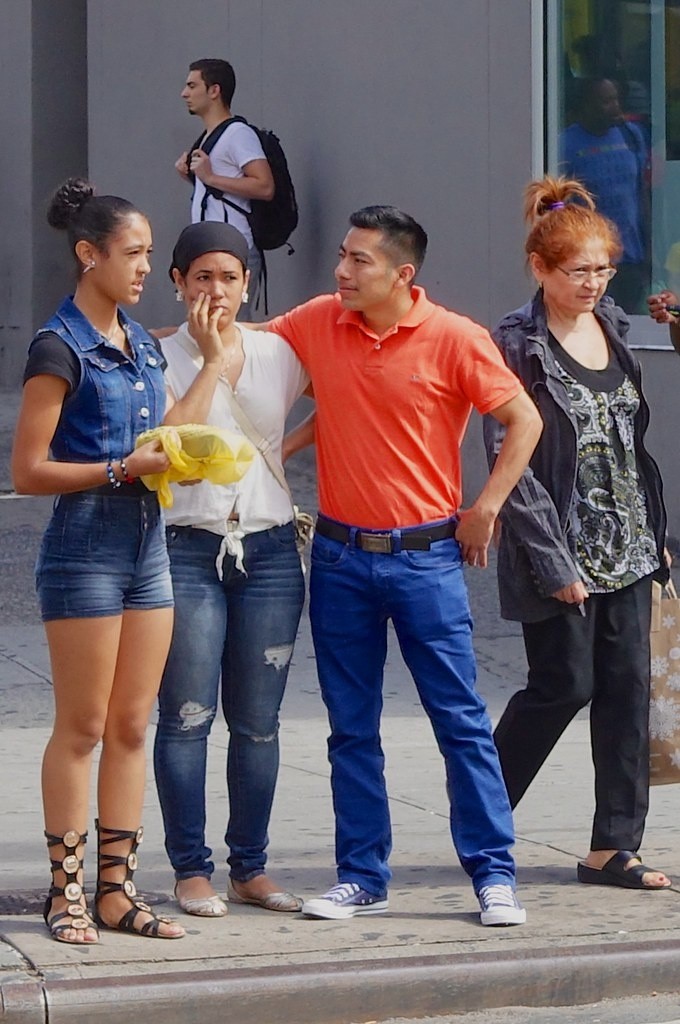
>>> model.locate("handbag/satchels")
[134,423,255,509]
[649,578,680,786]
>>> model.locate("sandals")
[92,818,185,939]
[174,880,228,917]
[227,875,304,912]
[43,830,99,944]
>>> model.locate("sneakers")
[478,884,526,926]
[301,883,389,919]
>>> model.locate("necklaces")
[221,327,236,376]
[109,324,119,342]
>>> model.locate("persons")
[13,182,200,946]
[147,204,543,925]
[483,177,671,891]
[152,219,315,919]
[176,59,274,323]
[559,53,651,313]
[647,290,680,355]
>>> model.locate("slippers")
[578,850,672,890]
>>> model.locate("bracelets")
[120,458,135,483]
[107,462,120,488]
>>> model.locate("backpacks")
[186,115,299,250]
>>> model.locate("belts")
[77,477,156,497]
[315,515,458,553]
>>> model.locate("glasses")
[555,262,619,284]
